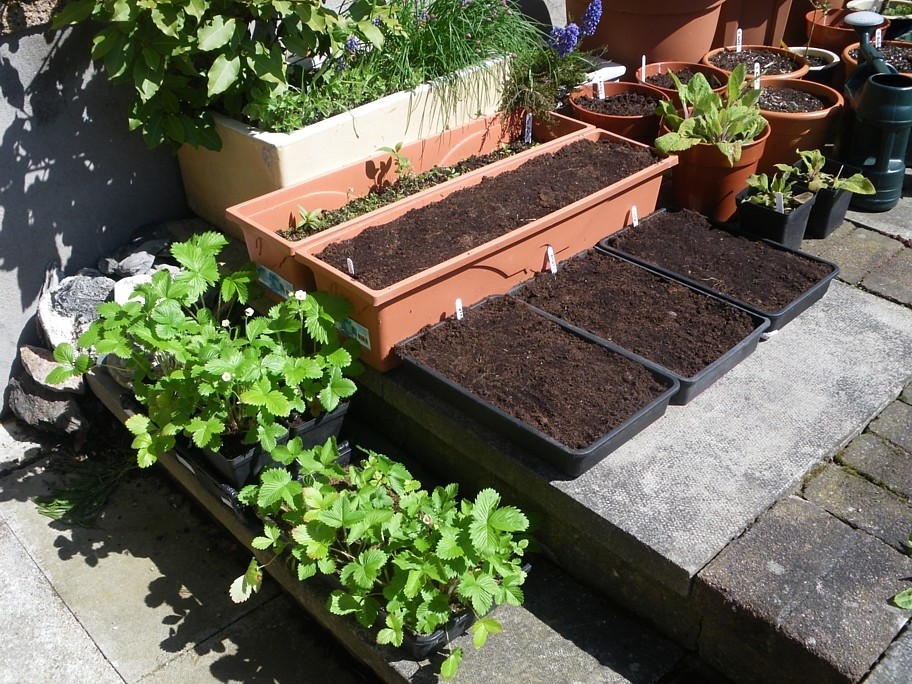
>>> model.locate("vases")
[162,49,508,239]
[298,0,912,477]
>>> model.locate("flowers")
[50,0,601,131]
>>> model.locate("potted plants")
[44,231,529,679]
[735,174,816,251]
[652,62,771,228]
[223,110,596,307]
[774,148,875,239]
